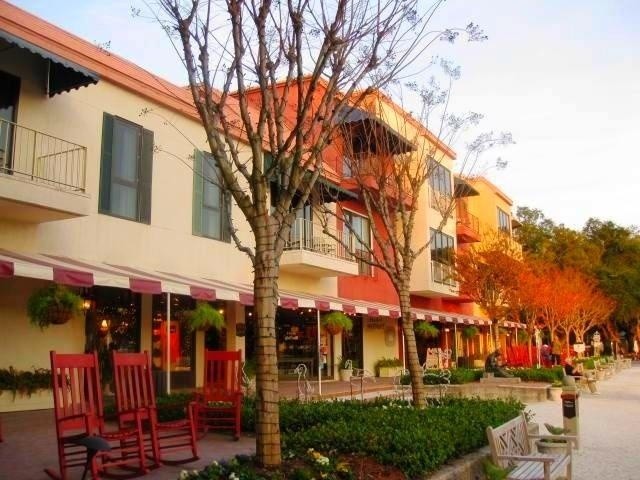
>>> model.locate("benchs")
[486,410,576,480]
[561,354,631,392]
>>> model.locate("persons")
[563,357,602,397]
[485,348,516,378]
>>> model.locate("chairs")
[191,348,244,440]
[48,348,149,479]
[110,348,201,467]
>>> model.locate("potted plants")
[375,357,403,377]
[25,281,82,333]
[178,300,227,334]
[413,320,440,340]
[463,325,480,342]
[320,310,354,335]
[338,354,354,382]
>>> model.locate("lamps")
[78,288,90,316]
[216,301,225,319]
